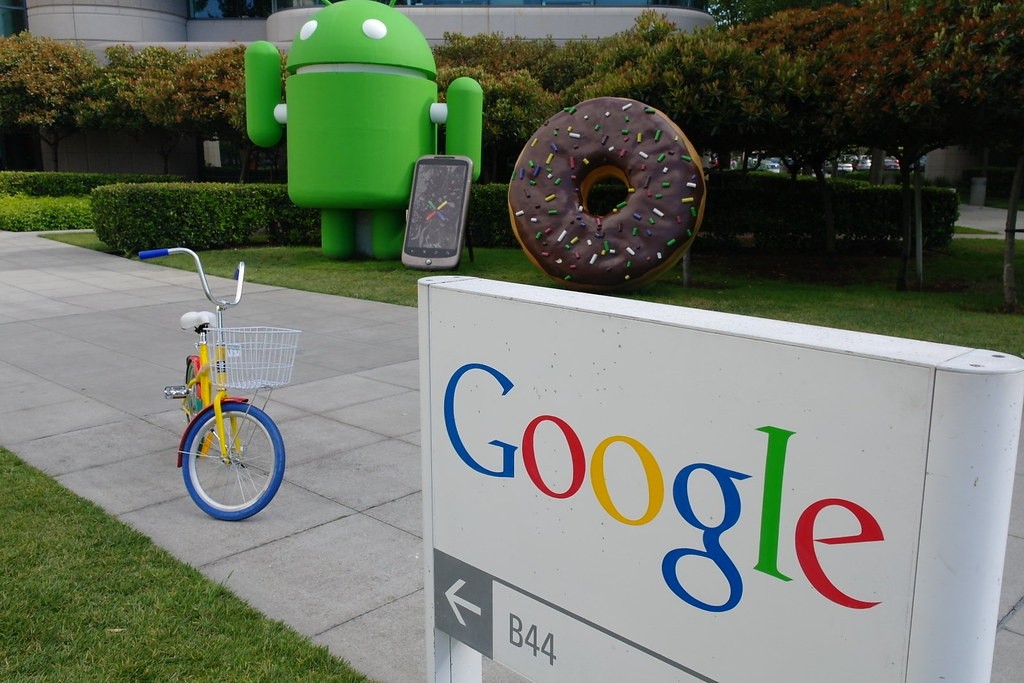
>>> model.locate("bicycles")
[138,248,304,522]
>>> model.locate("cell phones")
[401,155,473,272]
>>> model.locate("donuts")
[507,97,706,294]
